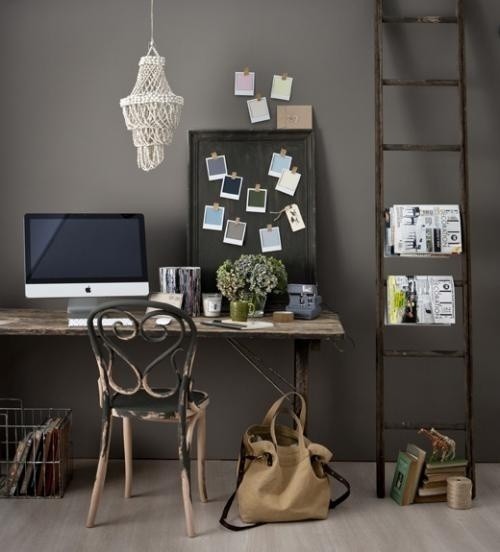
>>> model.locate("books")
[201,316,274,330]
[389,443,473,507]
[2,414,72,497]
[379,202,463,326]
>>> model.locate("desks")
[0,302,345,443]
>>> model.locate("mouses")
[156,317,172,326]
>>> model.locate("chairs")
[85,303,211,539]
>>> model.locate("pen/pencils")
[223,322,245,327]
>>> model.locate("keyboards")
[68,318,133,328]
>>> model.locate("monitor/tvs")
[23,212,150,313]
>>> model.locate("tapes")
[273,311,294,322]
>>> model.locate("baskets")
[0,398,73,500]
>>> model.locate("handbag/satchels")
[237,391,333,524]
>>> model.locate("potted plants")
[216,253,289,319]
